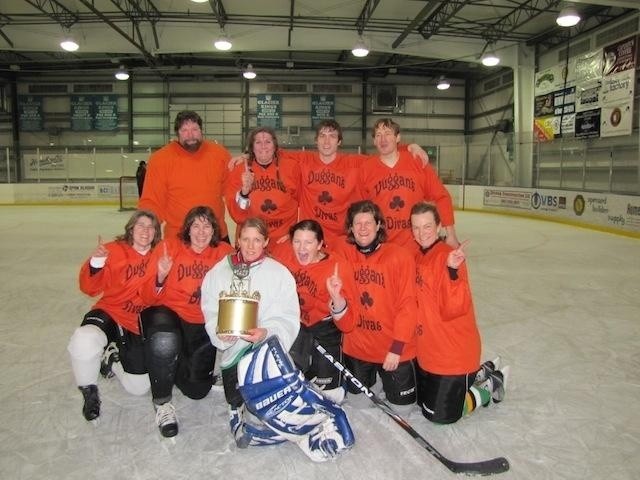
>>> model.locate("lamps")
[482,52,500,67]
[351,35,369,57]
[214,33,233,51]
[59,32,80,52]
[436,75,450,90]
[114,64,130,81]
[555,2,582,27]
[243,63,256,80]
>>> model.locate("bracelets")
[330,300,345,314]
[156,281,163,288]
[239,191,249,198]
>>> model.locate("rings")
[425,159,427,161]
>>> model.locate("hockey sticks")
[312,339,511,475]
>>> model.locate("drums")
[217,297,259,337]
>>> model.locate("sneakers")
[78,385,99,421]
[487,370,505,403]
[480,361,494,381]
[100,341,121,379]
[154,400,178,437]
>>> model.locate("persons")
[224,127,303,269]
[136,204,237,438]
[361,118,461,250]
[279,201,419,404]
[227,119,429,249]
[137,160,146,197]
[200,217,300,448]
[402,202,509,423]
[229,219,347,405]
[66,208,161,421]
[136,109,235,246]
